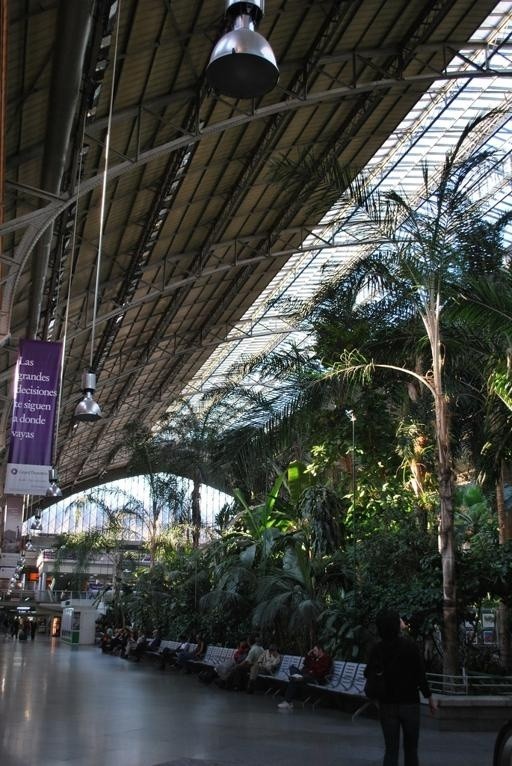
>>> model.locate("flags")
[2,336,65,499]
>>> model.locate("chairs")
[144,630,375,724]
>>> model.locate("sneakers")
[278,700,294,708]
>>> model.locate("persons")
[4,616,45,640]
[277,645,332,709]
[364,610,437,766]
[101,623,284,694]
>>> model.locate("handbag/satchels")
[362,651,387,699]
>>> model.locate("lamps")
[5,494,48,593]
[75,3,122,421]
[43,112,89,503]
[205,0,281,99]
